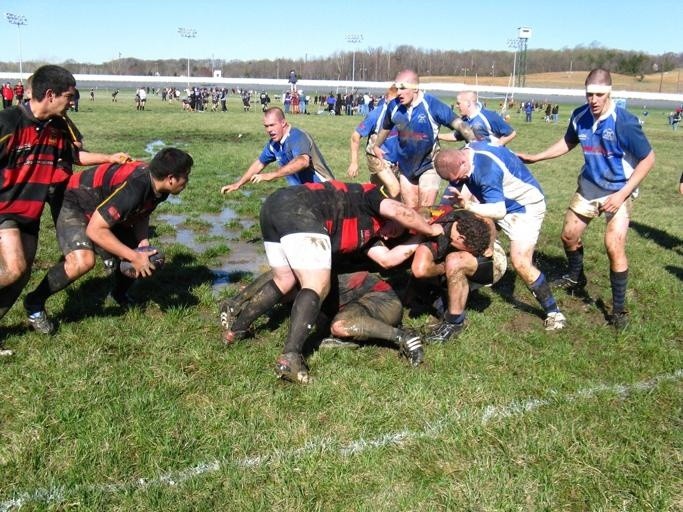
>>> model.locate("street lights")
[344,31,365,93]
[505,38,523,102]
[3,9,27,84]
[177,23,198,87]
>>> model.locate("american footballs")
[120,246,165,277]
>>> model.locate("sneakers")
[274,351,315,385]
[318,337,360,353]
[23,292,56,335]
[105,291,135,308]
[544,311,567,331]
[396,312,469,368]
[217,298,253,345]
[549,275,587,292]
[611,309,631,333]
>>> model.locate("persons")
[667,105,682,129]
[1,66,194,335]
[511,68,654,333]
[678,171,682,198]
[183,86,384,116]
[218,68,570,384]
[288,70,299,93]
